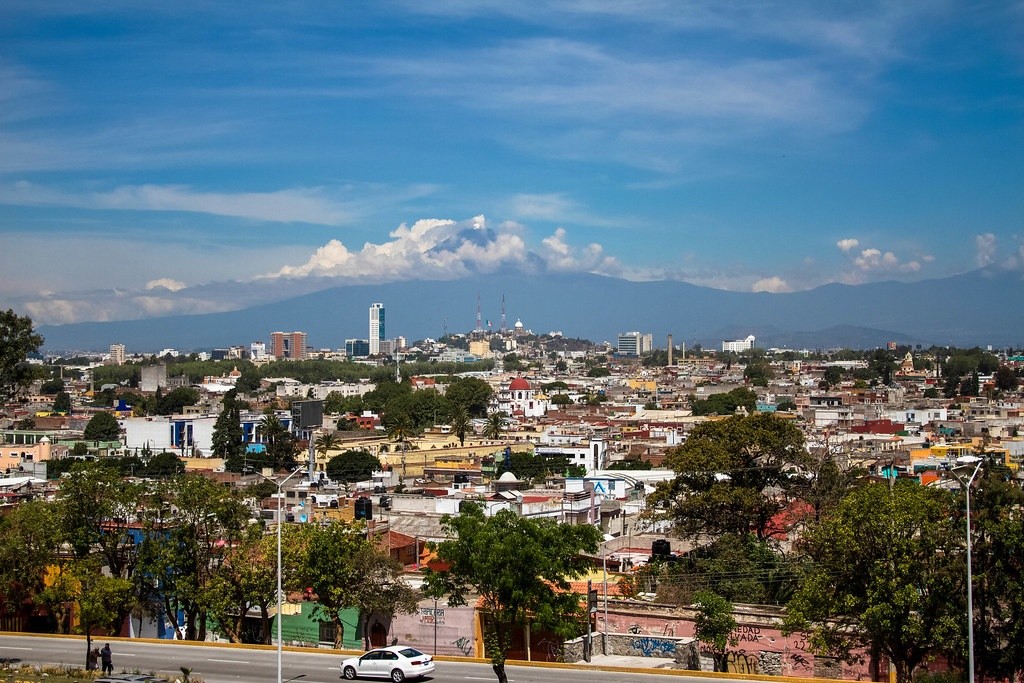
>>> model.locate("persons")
[100,643,114,676]
[88,645,105,670]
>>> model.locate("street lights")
[243,461,311,683]
[940,454,984,682]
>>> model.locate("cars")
[339,645,436,683]
